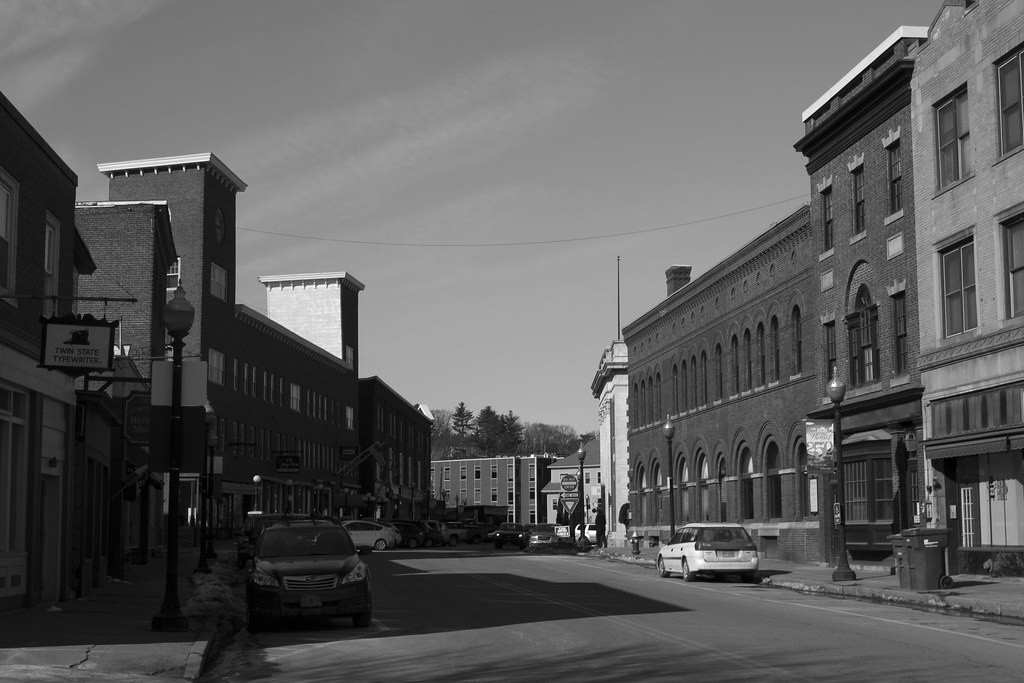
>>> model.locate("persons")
[595,507,607,548]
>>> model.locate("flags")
[369,444,390,466]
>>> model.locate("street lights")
[253,475,416,520]
[150,279,197,632]
[576,442,591,553]
[662,414,676,543]
[827,367,857,582]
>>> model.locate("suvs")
[495,522,528,551]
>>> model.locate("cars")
[575,524,597,546]
[655,522,759,583]
[339,519,499,551]
[529,526,559,544]
[245,517,372,634]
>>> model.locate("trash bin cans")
[887,534,902,586]
[901,528,954,590]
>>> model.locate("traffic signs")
[559,491,580,498]
